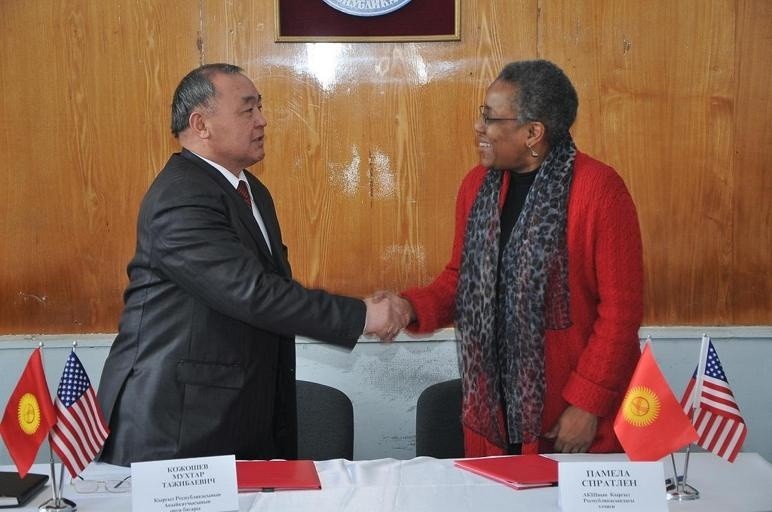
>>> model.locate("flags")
[0,343,59,481]
[612,342,701,463]
[678,333,749,464]
[46,348,111,482]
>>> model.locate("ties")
[235,181,253,212]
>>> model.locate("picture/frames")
[270,1,461,45]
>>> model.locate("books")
[451,453,559,491]
[235,459,323,494]
[0,471,51,509]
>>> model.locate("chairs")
[417,379,466,458]
[292,379,353,461]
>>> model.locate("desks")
[1,451,772,512]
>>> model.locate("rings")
[387,326,394,335]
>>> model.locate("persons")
[372,58,645,457]
[94,62,405,470]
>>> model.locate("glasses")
[479,104,522,124]
